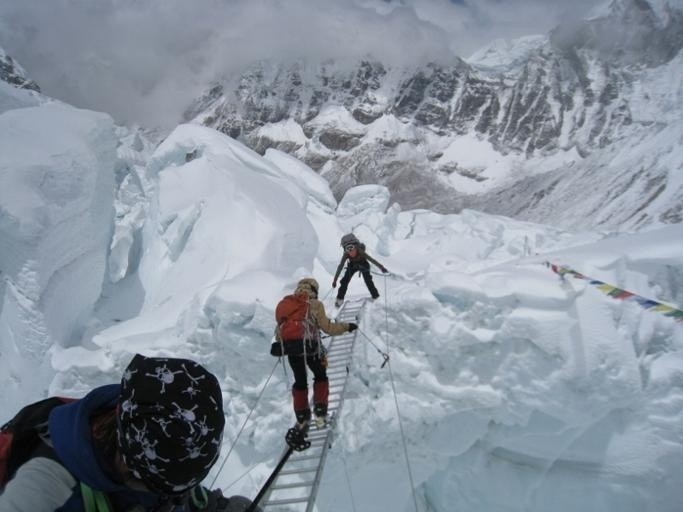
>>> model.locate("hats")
[298,278,319,293]
[118,353,224,494]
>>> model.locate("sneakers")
[335,298,344,306]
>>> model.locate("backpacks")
[271,291,318,357]
[341,233,359,248]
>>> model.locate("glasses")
[346,245,354,251]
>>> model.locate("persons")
[331,233,388,308]
[0,353,266,511]
[274,277,358,433]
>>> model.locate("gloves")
[348,323,357,332]
